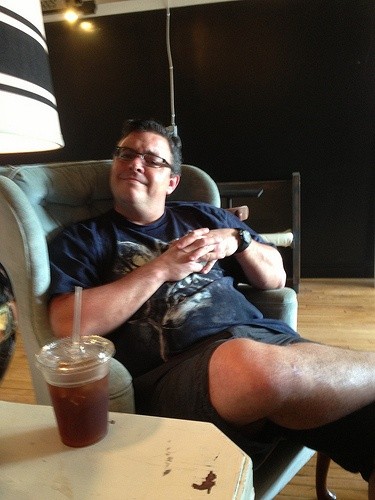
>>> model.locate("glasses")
[113,144,177,169]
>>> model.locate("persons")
[50,119,375,499]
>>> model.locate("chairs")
[0,158,338,500]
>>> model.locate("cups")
[36,335,116,447]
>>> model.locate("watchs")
[235,227,251,255]
